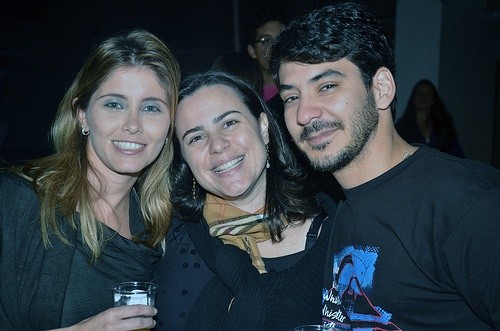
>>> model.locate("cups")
[111,282,159,319]
[294,324,337,331]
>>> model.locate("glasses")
[251,34,282,47]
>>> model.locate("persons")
[245,10,291,115]
[0,28,182,331]
[395,78,462,159]
[148,70,337,331]
[268,0,500,331]
